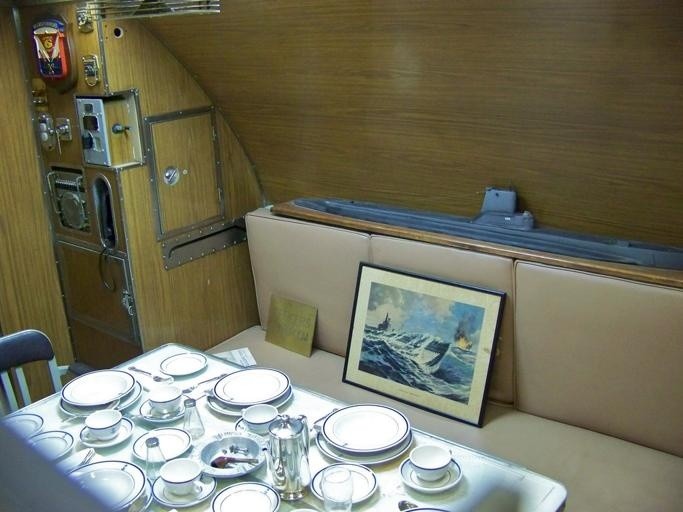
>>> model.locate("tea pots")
[260,414,310,501]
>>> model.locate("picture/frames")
[342,260,508,430]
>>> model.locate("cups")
[321,468,353,512]
[408,444,452,483]
[157,457,204,499]
[84,408,122,442]
[147,386,182,415]
[240,404,278,436]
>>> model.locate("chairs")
[1,329,65,414]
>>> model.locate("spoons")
[211,456,258,468]
[397,501,417,511]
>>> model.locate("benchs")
[206,190,682,511]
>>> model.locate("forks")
[129,366,169,383]
[78,418,134,448]
[183,374,226,394]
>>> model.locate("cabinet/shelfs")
[49,238,142,369]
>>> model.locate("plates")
[320,403,410,454]
[26,431,74,462]
[57,380,142,417]
[233,417,271,447]
[211,368,290,406]
[404,508,449,512]
[151,475,216,508]
[205,391,292,418]
[131,426,192,465]
[138,401,185,425]
[3,412,43,439]
[61,370,135,407]
[64,460,148,510]
[314,427,414,466]
[191,432,266,480]
[158,352,207,376]
[310,463,378,504]
[210,481,280,512]
[124,477,154,511]
[398,457,462,495]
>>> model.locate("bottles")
[184,399,207,448]
[145,437,166,483]
